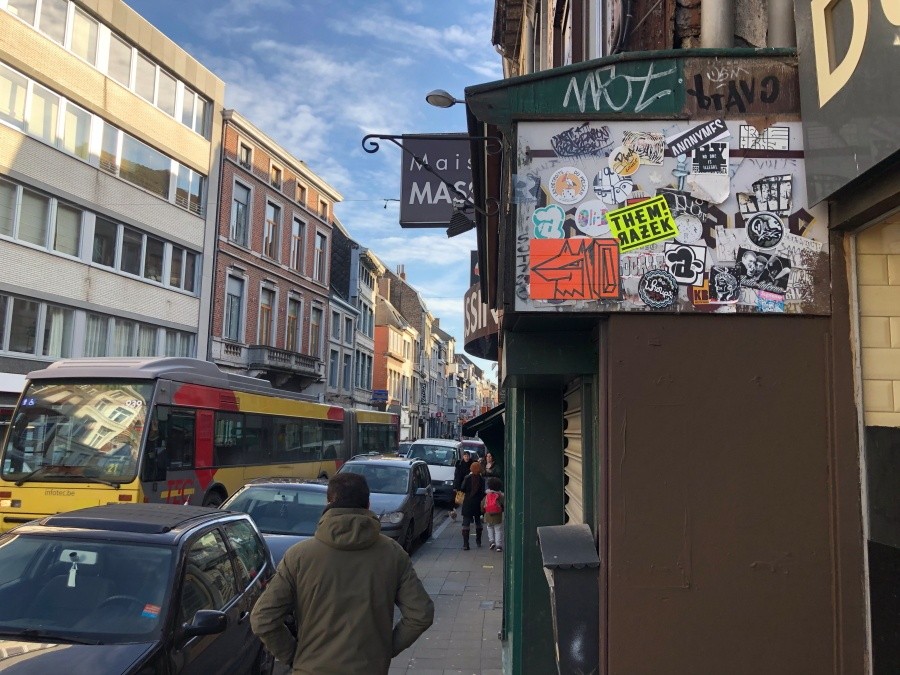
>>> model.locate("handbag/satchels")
[454,490,466,505]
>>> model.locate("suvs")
[0,503,284,675]
[336,451,436,559]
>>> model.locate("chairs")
[23,547,126,632]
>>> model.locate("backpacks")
[484,493,502,514]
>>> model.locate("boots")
[476,527,483,547]
[462,529,470,551]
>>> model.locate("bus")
[2,357,400,539]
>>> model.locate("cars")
[398,441,413,457]
[462,440,486,462]
[216,476,331,573]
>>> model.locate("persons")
[480,477,505,551]
[249,472,434,675]
[480,452,502,524]
[734,251,757,281]
[473,449,487,465]
[145,420,168,458]
[453,451,474,523]
[461,462,486,551]
[754,255,772,285]
[760,256,791,290]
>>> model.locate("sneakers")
[489,542,495,550]
[497,546,503,552]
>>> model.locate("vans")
[407,437,463,509]
